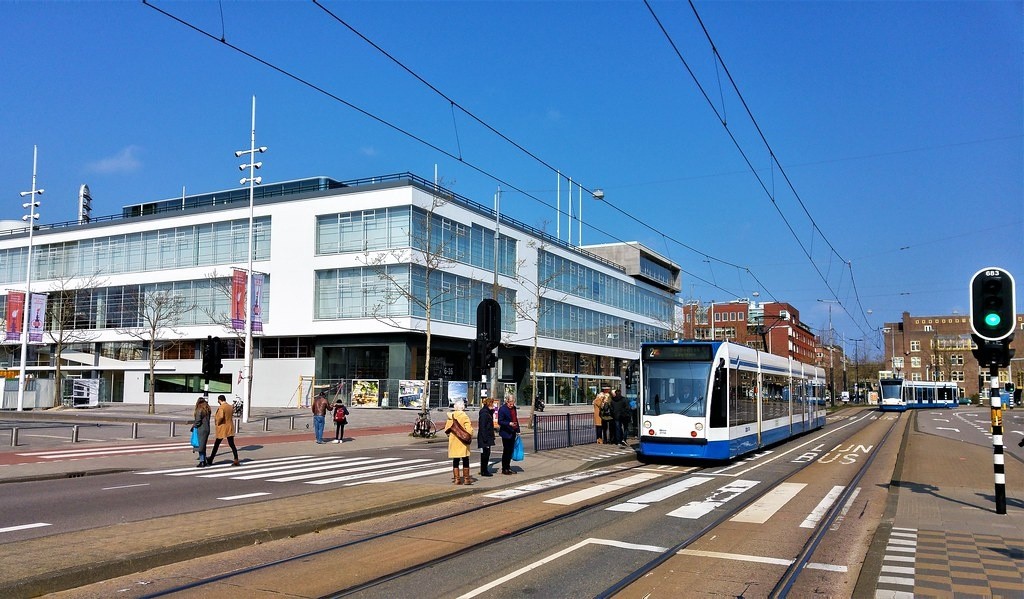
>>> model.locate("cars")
[960,397,973,406]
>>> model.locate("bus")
[783,384,826,405]
[877,367,960,411]
[624,313,828,461]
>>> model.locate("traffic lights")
[979,375,984,386]
[969,266,1017,340]
[467,340,481,367]
[1004,382,1017,392]
[999,334,1017,368]
[490,353,499,368]
[969,333,987,367]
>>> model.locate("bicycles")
[413,408,438,439]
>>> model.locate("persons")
[498,395,521,475]
[312,392,333,444]
[477,397,496,477]
[676,385,698,403]
[206,395,241,466]
[444,401,474,485]
[190,397,211,467]
[332,399,349,444]
[592,389,640,448]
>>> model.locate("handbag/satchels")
[450,414,473,444]
[190,427,200,448]
[598,407,604,418]
[512,434,524,461]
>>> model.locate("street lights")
[233,92,268,425]
[849,338,864,403]
[817,298,842,408]
[17,145,45,412]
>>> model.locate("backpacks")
[602,399,611,416]
[335,407,345,422]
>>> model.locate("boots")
[462,467,471,485]
[454,467,463,485]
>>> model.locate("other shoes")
[482,471,493,476]
[618,444,625,449]
[596,438,604,444]
[318,440,326,444]
[206,457,212,466]
[230,458,239,466]
[340,439,343,443]
[332,439,338,443]
[622,440,628,446]
[196,461,205,467]
[502,468,517,475]
